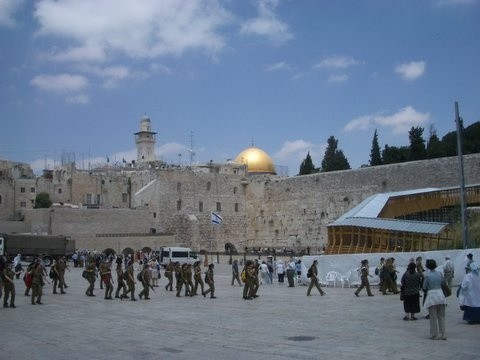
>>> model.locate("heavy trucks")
[0,232,75,267]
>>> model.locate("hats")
[467,253,473,260]
[278,260,282,264]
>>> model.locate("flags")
[211,212,223,225]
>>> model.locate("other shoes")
[10,304,15,308]
[85,293,151,301]
[380,288,400,296]
[244,294,258,300]
[354,292,359,297]
[165,287,216,299]
[3,303,9,308]
[368,294,373,297]
[403,316,417,320]
[429,335,447,340]
[24,291,66,305]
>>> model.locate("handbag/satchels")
[375,268,379,275]
[440,272,451,298]
[283,270,285,275]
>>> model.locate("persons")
[296,260,302,275]
[243,259,273,300]
[409,256,425,289]
[276,260,285,282]
[72,252,160,301]
[287,258,296,287]
[23,256,70,305]
[422,259,448,340]
[2,260,23,308]
[401,263,420,321]
[354,260,374,296]
[231,259,241,286]
[165,261,217,298]
[307,260,326,296]
[458,262,480,325]
[14,253,22,279]
[442,255,455,293]
[465,254,473,274]
[378,257,400,295]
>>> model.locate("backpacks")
[49,264,57,278]
[124,271,128,280]
[137,270,145,281]
[26,272,32,287]
[357,267,366,277]
[306,264,315,278]
[241,268,247,282]
[82,270,88,278]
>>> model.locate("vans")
[159,246,200,266]
